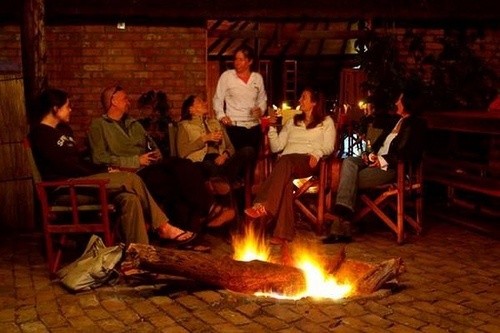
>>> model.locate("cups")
[215,128,223,146]
[249,107,257,118]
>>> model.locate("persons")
[27,45,430,250]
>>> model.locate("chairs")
[21,123,424,280]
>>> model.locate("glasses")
[110,86,122,103]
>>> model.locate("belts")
[232,121,258,129]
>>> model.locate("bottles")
[366,140,376,165]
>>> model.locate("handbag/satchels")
[55,235,124,291]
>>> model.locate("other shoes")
[205,178,230,194]
[329,204,357,223]
[270,237,292,245]
[207,204,234,228]
[321,233,352,243]
[245,204,269,217]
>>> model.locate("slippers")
[158,229,197,246]
